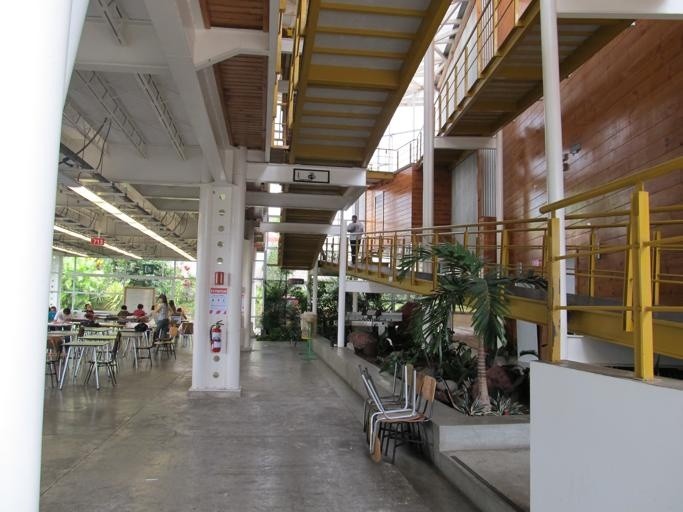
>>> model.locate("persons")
[362,305,368,314]
[348,214,364,264]
[374,307,381,318]
[47,293,186,353]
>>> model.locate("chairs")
[358,363,435,466]
[154,312,193,359]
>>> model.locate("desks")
[46,313,159,390]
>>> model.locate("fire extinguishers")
[209,320,224,352]
